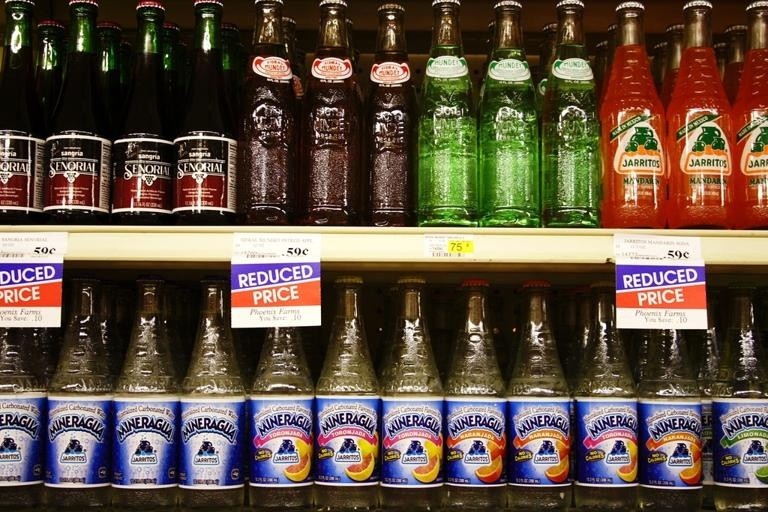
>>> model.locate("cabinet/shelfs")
[0,0,768,512]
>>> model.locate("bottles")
[0,263,768,512]
[0,0,768,228]
